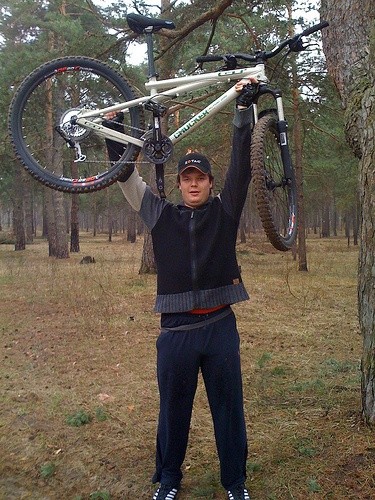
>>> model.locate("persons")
[98,76,264,500]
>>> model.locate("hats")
[178,154,211,175]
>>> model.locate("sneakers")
[153,482,180,500]
[227,489,251,500]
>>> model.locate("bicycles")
[7,12,329,252]
